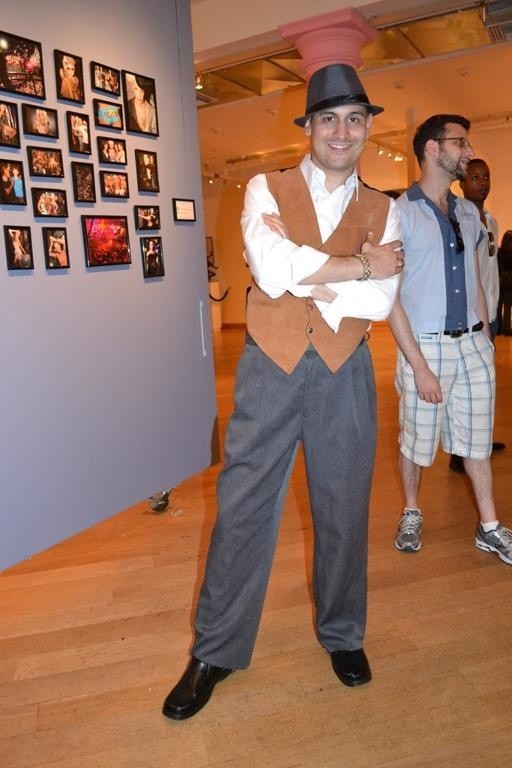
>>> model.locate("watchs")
[353,253,373,281]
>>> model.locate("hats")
[294,64,384,127]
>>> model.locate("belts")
[428,321,484,338]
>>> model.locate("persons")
[25,103,58,138]
[103,171,129,197]
[135,151,158,192]
[448,157,512,473]
[388,114,512,563]
[161,61,406,720]
[497,230,511,335]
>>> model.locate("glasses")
[435,137,471,149]
[449,219,465,254]
[488,232,495,256]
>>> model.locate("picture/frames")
[0,100,21,149]
[99,169,129,198]
[66,110,92,155]
[30,186,68,218]
[172,197,196,221]
[89,60,121,96]
[134,148,160,192]
[133,204,160,230]
[96,135,128,165]
[21,102,59,139]
[26,145,65,178]
[92,98,124,130]
[0,30,46,100]
[80,214,132,267]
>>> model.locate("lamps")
[378,145,384,155]
[395,155,403,160]
[388,151,392,157]
[195,75,203,89]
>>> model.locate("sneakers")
[394,508,423,551]
[475,522,512,565]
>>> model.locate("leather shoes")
[491,442,506,450]
[332,648,372,687]
[163,656,234,720]
[450,455,465,473]
